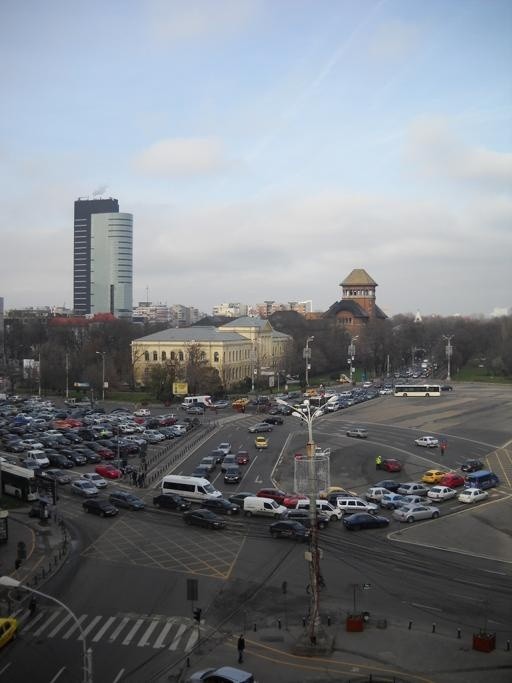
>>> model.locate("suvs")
[186,401,206,411]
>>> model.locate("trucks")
[181,395,212,410]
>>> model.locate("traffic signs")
[362,582,372,590]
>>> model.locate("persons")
[119,441,147,488]
[29,597,39,618]
[376,456,382,470]
[14,555,24,581]
[440,439,448,455]
[237,634,247,664]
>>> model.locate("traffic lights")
[193,607,202,622]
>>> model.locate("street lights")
[96,350,106,400]
[305,335,315,386]
[442,334,455,380]
[65,349,71,396]
[349,335,360,381]
[301,299,313,312]
[274,393,341,627]
[0,576,94,681]
[38,348,43,394]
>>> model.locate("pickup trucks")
[232,397,250,408]
[74,380,90,387]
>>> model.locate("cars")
[109,491,146,511]
[201,498,240,515]
[376,458,401,473]
[1,617,17,648]
[95,463,123,479]
[80,472,108,490]
[248,422,274,433]
[249,387,379,417]
[184,508,226,530]
[187,406,204,415]
[83,499,119,517]
[386,383,394,388]
[390,358,440,380]
[45,469,72,485]
[375,382,385,388]
[213,399,228,408]
[189,665,256,683]
[462,458,482,471]
[345,427,368,439]
[255,436,269,449]
[0,392,191,469]
[69,480,99,498]
[153,494,192,511]
[414,435,440,447]
[192,443,250,485]
[363,381,373,388]
[440,385,453,391]
[163,474,223,504]
[230,469,501,544]
[264,415,284,425]
[377,389,393,395]
